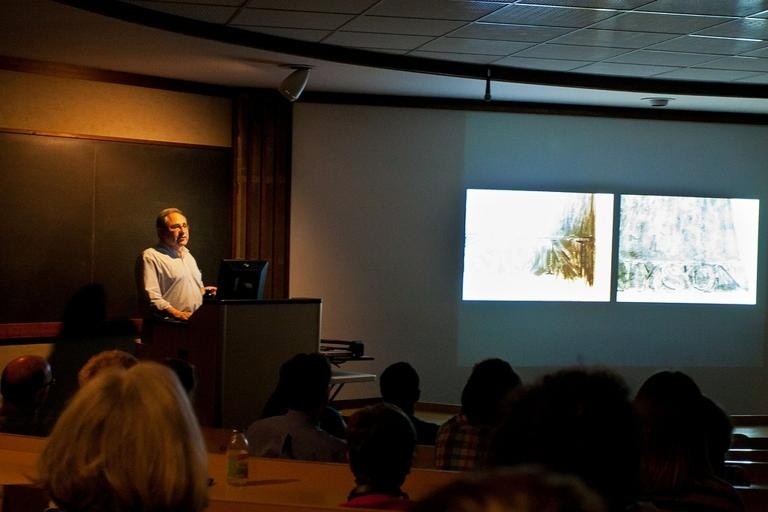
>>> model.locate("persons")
[135,205,219,346]
[2,346,752,510]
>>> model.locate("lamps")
[278,68,311,102]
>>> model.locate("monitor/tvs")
[216,258,269,299]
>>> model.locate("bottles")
[228,428,250,485]
